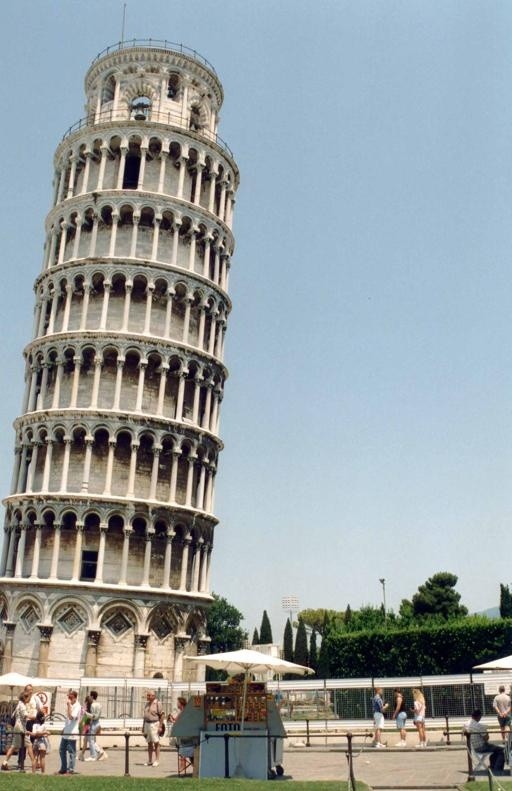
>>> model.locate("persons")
[2,685,194,774]
[409,687,425,744]
[275,690,282,702]
[392,688,407,747]
[464,708,505,775]
[492,686,511,742]
[371,688,389,748]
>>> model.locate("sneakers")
[1,763,41,771]
[488,768,510,776]
[142,761,160,767]
[53,769,74,776]
[78,751,108,762]
[373,740,427,748]
[498,742,508,748]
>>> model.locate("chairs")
[459,723,508,782]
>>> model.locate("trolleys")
[201,678,288,781]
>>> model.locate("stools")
[176,750,195,777]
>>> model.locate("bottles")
[246,696,267,722]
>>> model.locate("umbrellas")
[183,648,315,731]
[472,655,512,670]
[0,671,59,705]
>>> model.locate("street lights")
[378,576,389,630]
[280,594,301,621]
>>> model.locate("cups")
[215,723,240,731]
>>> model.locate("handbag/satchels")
[159,723,165,737]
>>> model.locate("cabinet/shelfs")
[202,681,272,734]
